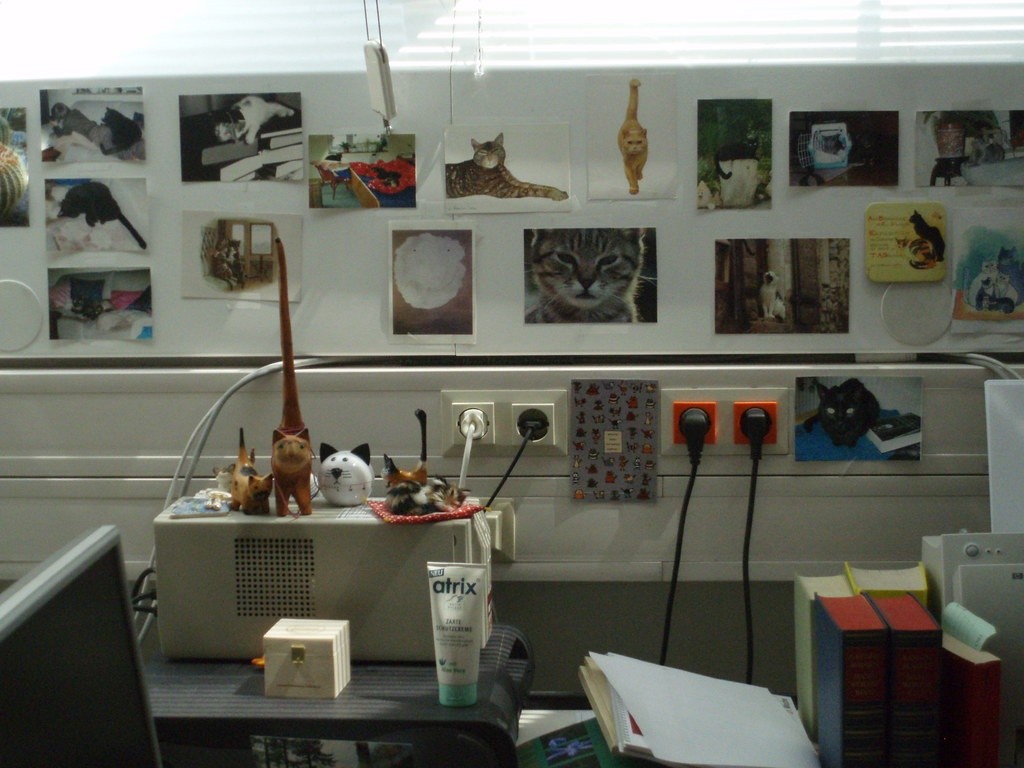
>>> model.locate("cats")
[804,378,880,449]
[962,245,1024,314]
[974,142,1005,165]
[325,152,342,161]
[211,237,245,292]
[385,474,472,516]
[212,449,256,496]
[909,209,946,262]
[99,107,143,156]
[70,293,103,322]
[525,227,648,323]
[760,268,786,323]
[617,79,648,195]
[821,133,847,156]
[895,236,938,270]
[270,235,313,516]
[714,135,760,180]
[207,95,294,145]
[58,182,146,250]
[446,132,569,202]
[228,427,274,515]
[380,409,429,490]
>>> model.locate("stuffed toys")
[385,477,466,518]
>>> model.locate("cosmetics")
[422,559,490,711]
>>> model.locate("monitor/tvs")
[0,523,164,768]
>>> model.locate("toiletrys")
[426,559,488,708]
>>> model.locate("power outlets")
[658,386,792,458]
[437,387,569,459]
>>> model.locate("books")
[573,642,826,768]
[810,588,891,767]
[843,557,933,613]
[860,587,947,765]
[936,625,1004,768]
[788,568,859,742]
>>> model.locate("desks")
[0,575,536,767]
